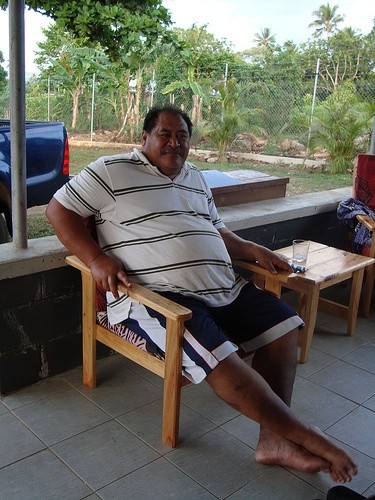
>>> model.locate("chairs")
[353,155,375,321]
[65,254,299,448]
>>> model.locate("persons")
[45,105,358,483]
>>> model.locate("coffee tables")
[257,241,373,362]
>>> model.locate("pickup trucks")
[0,118,71,243]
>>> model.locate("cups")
[293,240,310,271]
